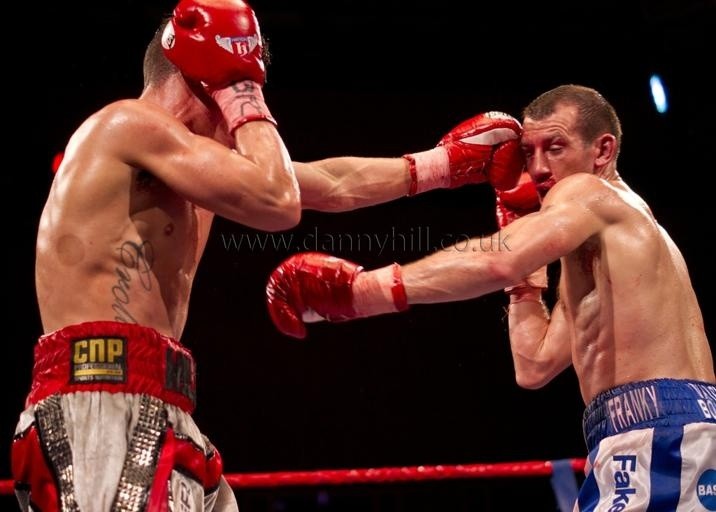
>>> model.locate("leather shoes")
[401,110,523,197]
[494,169,549,294]
[265,251,411,341]
[159,0,279,148]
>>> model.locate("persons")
[11,0,523,512]
[265,84,716,512]
